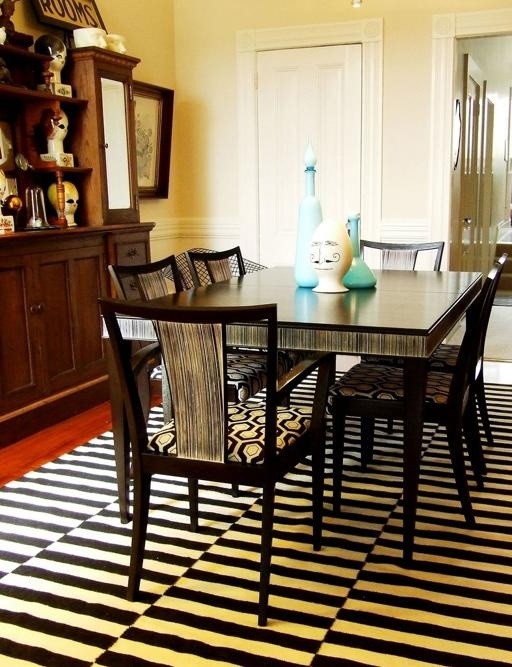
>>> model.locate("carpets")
[0,381,512,667]
[446,301,511,364]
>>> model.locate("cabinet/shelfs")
[0,41,157,450]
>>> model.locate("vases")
[342,215,377,290]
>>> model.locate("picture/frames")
[131,78,175,200]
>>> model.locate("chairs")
[359,253,511,477]
[359,239,448,272]
[93,295,333,627]
[106,254,291,529]
[183,246,319,401]
[325,265,499,528]
